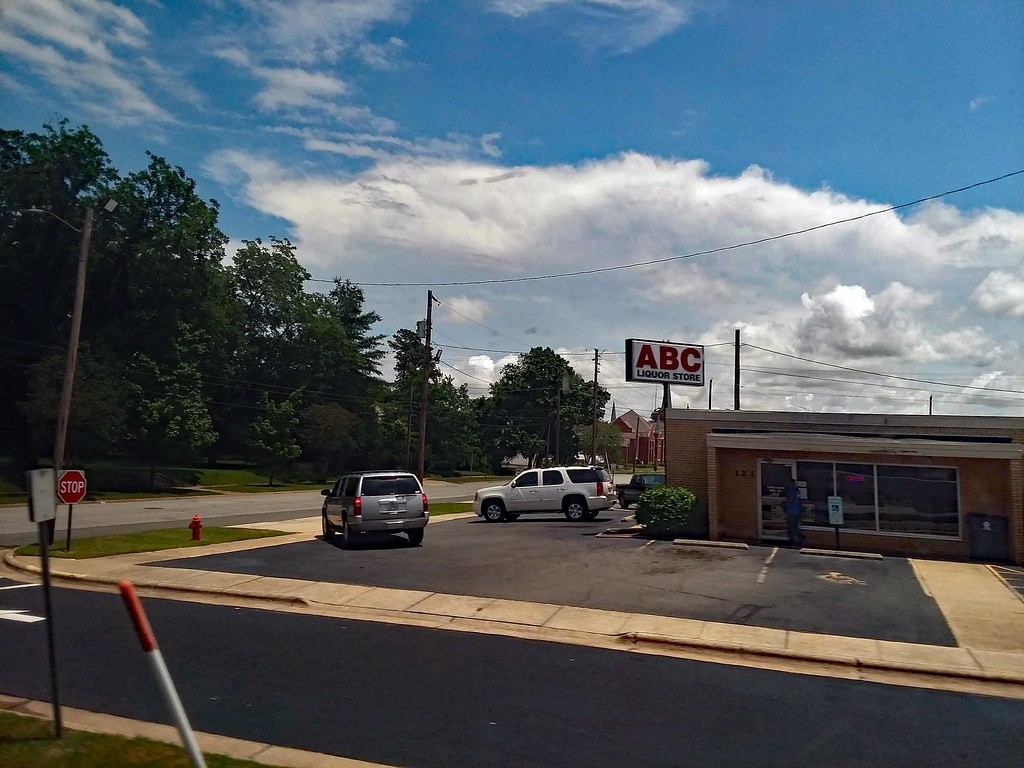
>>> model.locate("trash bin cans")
[968,512,1010,561]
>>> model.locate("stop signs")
[58,471,87,504]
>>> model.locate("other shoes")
[796,535,806,544]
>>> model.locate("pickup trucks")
[615,472,666,508]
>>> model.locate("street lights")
[417,349,444,487]
[25,199,120,543]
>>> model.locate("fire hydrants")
[188,514,202,540]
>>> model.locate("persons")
[782,480,806,547]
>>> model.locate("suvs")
[321,471,429,548]
[472,464,619,522]
